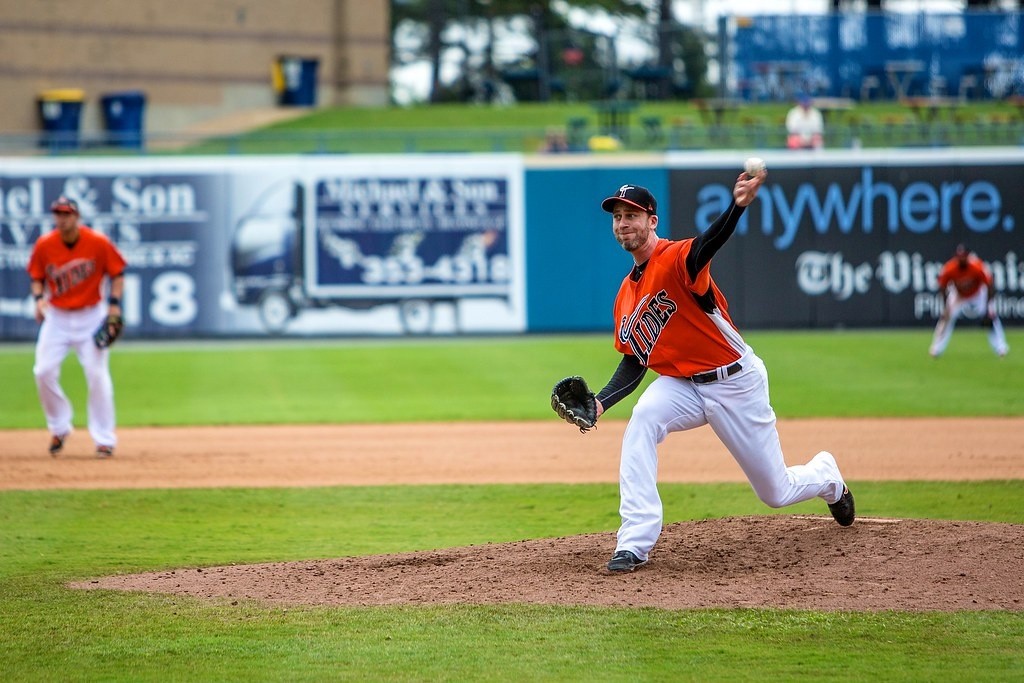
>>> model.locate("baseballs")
[744,157,766,176]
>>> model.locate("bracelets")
[108,297,117,306]
[34,294,43,301]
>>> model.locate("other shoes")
[97,445,112,456]
[50,431,69,454]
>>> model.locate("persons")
[454,44,1024,151]
[27,196,128,456]
[927,244,1008,358]
[550,169,854,571]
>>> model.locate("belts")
[53,307,96,314]
[685,363,743,383]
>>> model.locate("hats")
[49,197,79,215]
[601,185,657,215]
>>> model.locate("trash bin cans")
[37,89,85,148]
[271,53,320,106]
[100,89,145,148]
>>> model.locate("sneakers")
[827,481,856,526]
[607,550,648,574]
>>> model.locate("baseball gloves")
[94,314,123,349]
[550,373,600,433]
